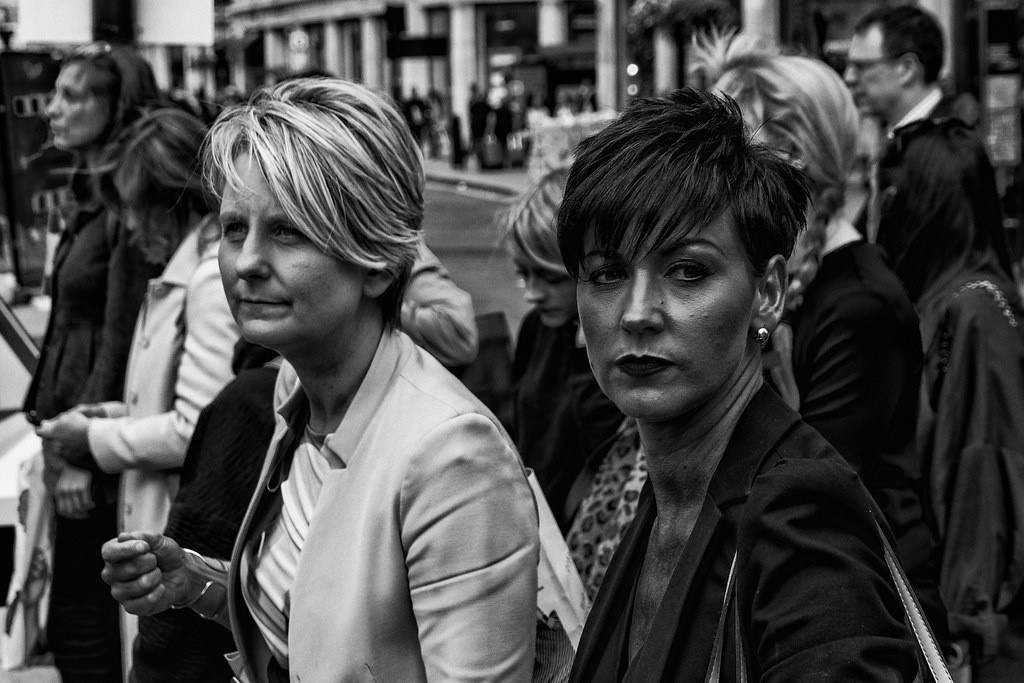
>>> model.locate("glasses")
[73,40,116,71]
[846,47,910,73]
[893,114,968,161]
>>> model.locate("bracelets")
[171,549,229,619]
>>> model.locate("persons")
[20,6,1024,683]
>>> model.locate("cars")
[0,298,53,672]
